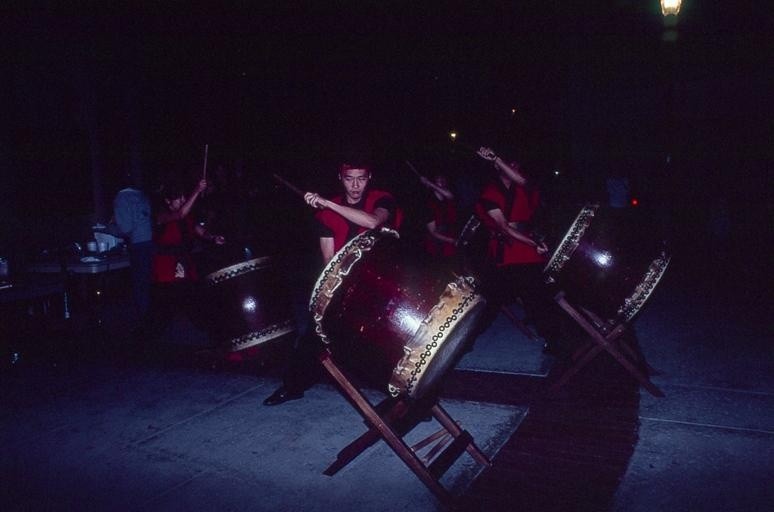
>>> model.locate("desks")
[25,254,133,334]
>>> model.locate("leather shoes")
[263,386,305,406]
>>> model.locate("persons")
[106,170,153,325]
[151,173,207,285]
[473,144,579,340]
[261,147,402,406]
[418,166,456,257]
[197,160,261,240]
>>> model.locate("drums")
[204,255,294,355]
[544,201,670,321]
[308,227,486,404]
